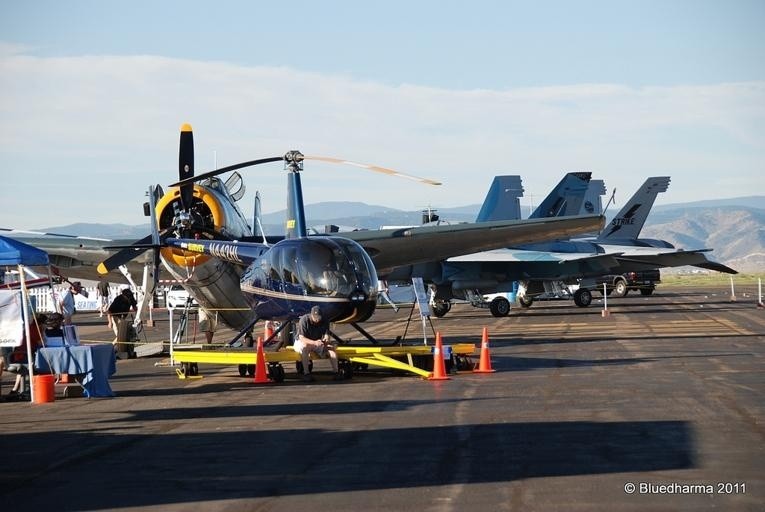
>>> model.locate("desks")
[34,343,117,399]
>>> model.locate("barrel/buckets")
[33,374,54,404]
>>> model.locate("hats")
[311,306,322,321]
[123,288,133,297]
[73,282,84,289]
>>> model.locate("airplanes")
[0,123,739,386]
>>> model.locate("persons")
[106,289,137,345]
[294,306,346,382]
[96,275,112,317]
[198,306,217,345]
[56,282,82,326]
[45,312,64,337]
[12,314,49,363]
